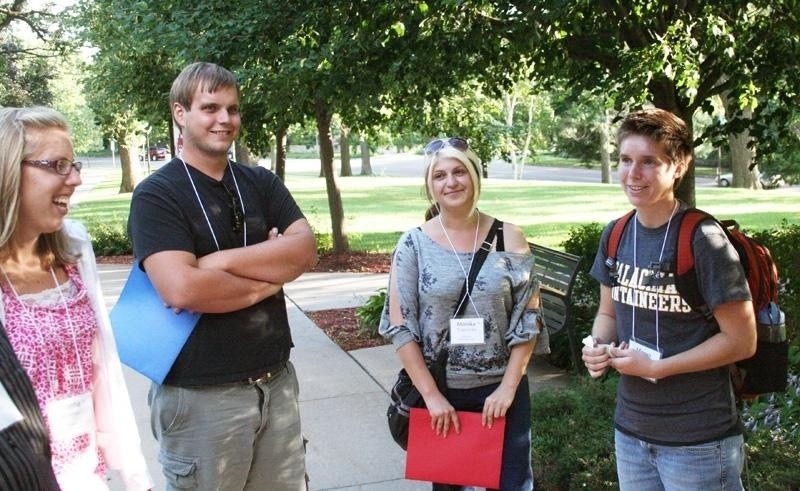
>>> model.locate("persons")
[0,106,157,490]
[126,61,317,491]
[378,137,553,491]
[578,104,757,489]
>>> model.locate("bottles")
[760,302,787,341]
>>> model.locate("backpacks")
[604,205,790,403]
[385,348,455,452]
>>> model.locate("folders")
[107,261,205,386]
[404,408,506,490]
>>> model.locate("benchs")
[528,240,585,375]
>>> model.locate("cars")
[716,166,784,190]
[137,145,166,162]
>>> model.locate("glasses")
[423,137,472,158]
[20,157,83,176]
